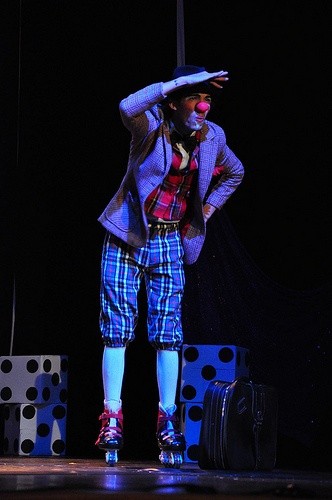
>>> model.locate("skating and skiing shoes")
[95,398,125,466]
[156,404,187,470]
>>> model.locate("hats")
[166,65,217,102]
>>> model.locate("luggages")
[197,377,266,473]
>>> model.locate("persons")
[93,65,245,468]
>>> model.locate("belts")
[146,215,181,224]
